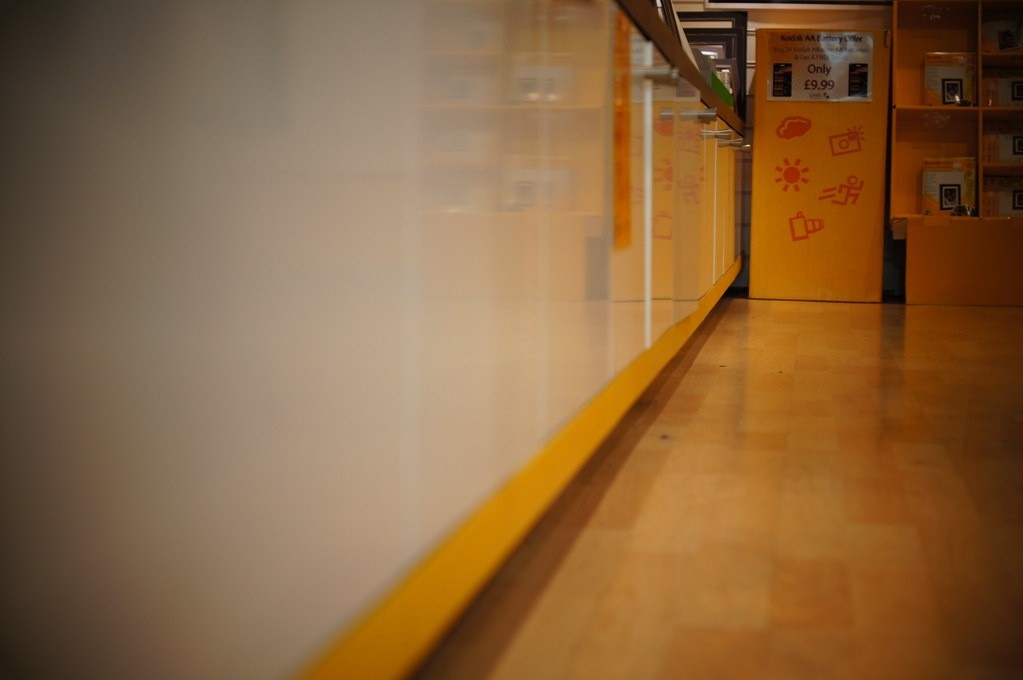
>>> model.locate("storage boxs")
[750,28,888,301]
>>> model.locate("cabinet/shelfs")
[889,0,1023,224]
